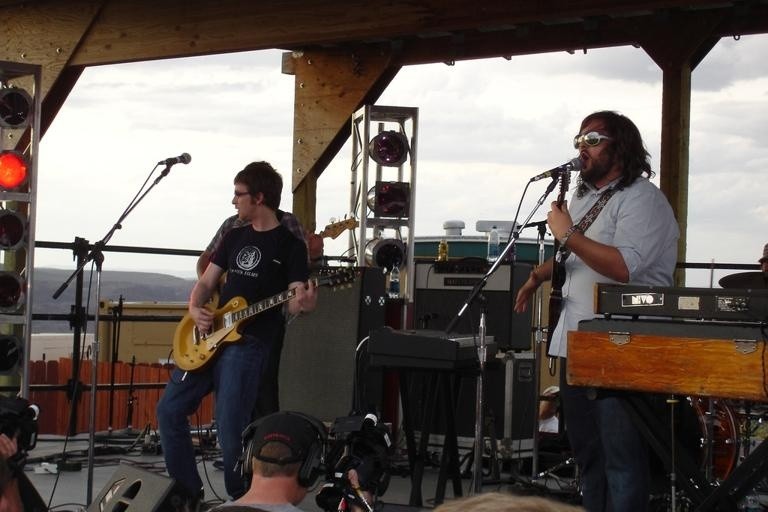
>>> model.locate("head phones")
[237,411,328,488]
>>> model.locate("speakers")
[88,461,184,512]
[278,266,387,427]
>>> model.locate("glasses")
[234,191,250,199]
[572,131,609,150]
[486,479,578,510]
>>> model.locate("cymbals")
[719,272,768,292]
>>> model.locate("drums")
[650,395,741,495]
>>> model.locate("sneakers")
[173,493,200,511]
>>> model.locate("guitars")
[173,266,356,371]
[546,165,571,358]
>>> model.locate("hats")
[251,413,315,465]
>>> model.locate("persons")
[196,206,325,435]
[1,391,30,512]
[155,162,318,512]
[207,410,332,512]
[513,110,680,511]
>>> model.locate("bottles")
[486,224,500,263]
[437,238,448,262]
[387,262,400,299]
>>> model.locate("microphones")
[532,157,584,184]
[158,153,193,166]
